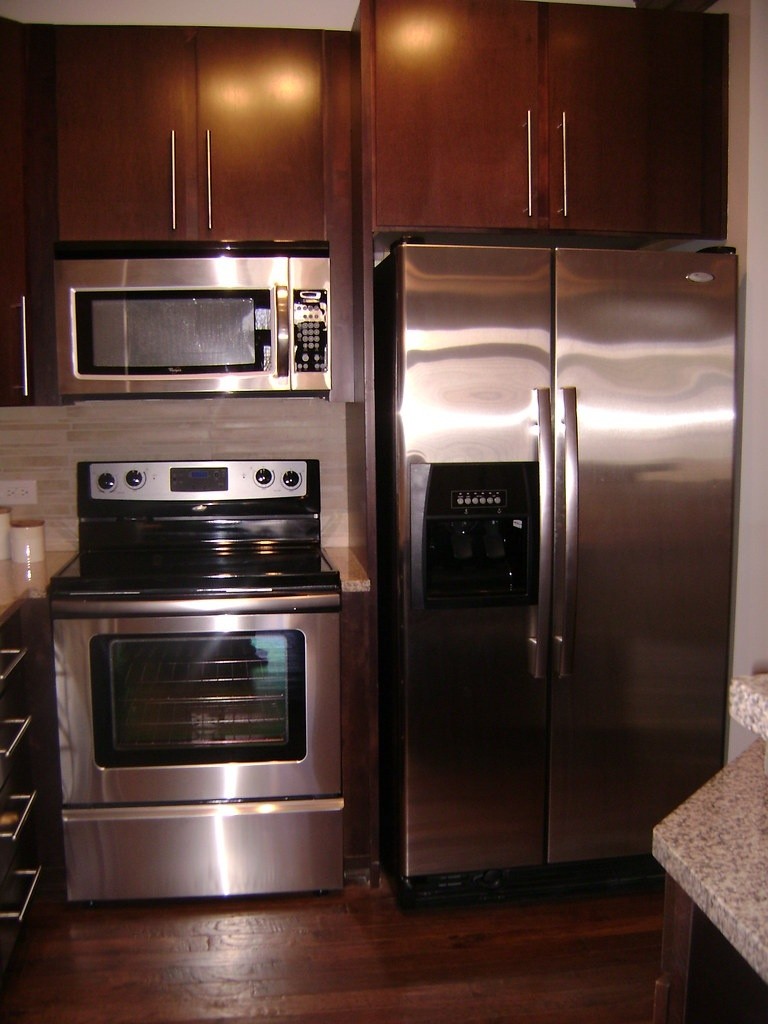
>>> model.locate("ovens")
[48,595,345,907]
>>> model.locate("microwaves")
[51,236,332,398]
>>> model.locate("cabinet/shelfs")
[356,0,728,246]
[56,24,328,244]
[0,550,82,977]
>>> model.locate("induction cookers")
[49,460,342,589]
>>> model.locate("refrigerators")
[367,240,741,906]
